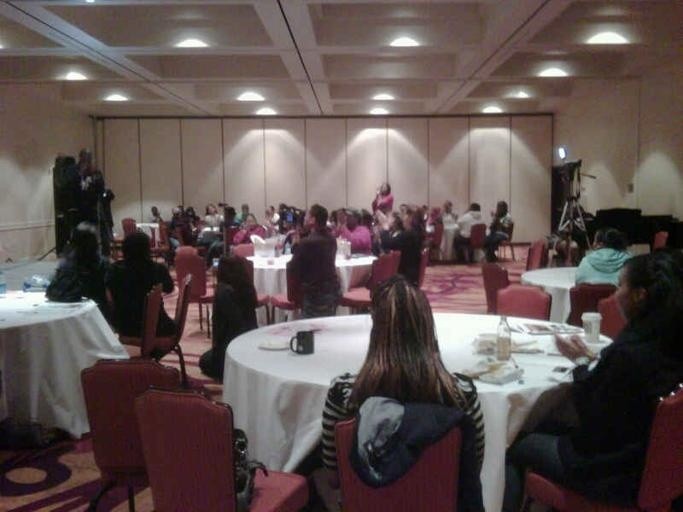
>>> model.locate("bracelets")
[571,355,592,366]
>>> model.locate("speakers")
[55,156,76,257]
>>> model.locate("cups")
[580,311,603,345]
[288,330,314,355]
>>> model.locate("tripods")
[546,185,592,268]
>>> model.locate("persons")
[373,205,429,293]
[372,181,394,213]
[208,253,261,381]
[573,226,638,296]
[282,203,342,321]
[390,199,515,264]
[327,207,383,254]
[501,250,682,512]
[149,203,286,258]
[43,218,105,319]
[51,147,114,257]
[320,272,488,512]
[90,231,180,362]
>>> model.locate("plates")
[257,340,288,351]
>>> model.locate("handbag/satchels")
[46,271,83,302]
[232,428,268,512]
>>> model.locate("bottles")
[0,272,7,299]
[496,312,511,361]
